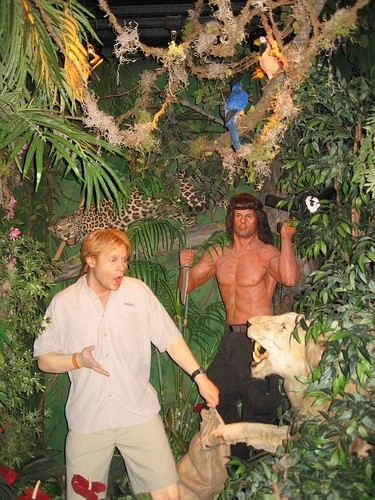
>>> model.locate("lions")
[200,312,370,461]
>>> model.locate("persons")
[32,229,220,500]
[178,193,298,462]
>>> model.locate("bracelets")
[72,352,80,369]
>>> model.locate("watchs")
[192,367,207,381]
